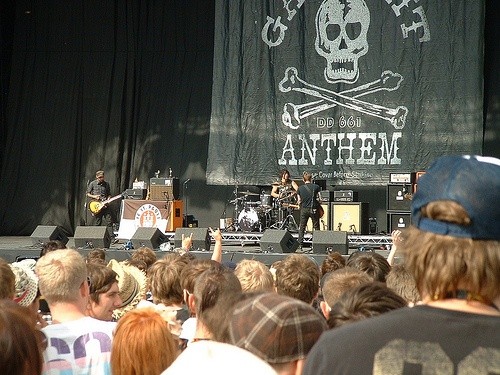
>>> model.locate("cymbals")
[270,182,284,187]
[238,192,260,196]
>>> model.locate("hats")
[104,258,147,319]
[225,293,328,363]
[410,154,500,243]
[7,261,39,309]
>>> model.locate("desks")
[119,200,183,232]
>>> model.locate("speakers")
[130,227,170,251]
[305,201,329,232]
[386,183,414,212]
[29,225,69,247]
[149,177,179,200]
[389,213,411,233]
[313,230,348,255]
[125,189,146,200]
[331,200,370,235]
[260,230,300,253]
[74,226,111,250]
[174,228,211,252]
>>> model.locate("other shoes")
[298,246,302,250]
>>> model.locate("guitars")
[291,180,324,218]
[87,191,125,216]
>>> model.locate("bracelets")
[185,248,189,252]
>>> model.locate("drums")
[237,206,271,231]
[259,195,274,209]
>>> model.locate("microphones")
[183,179,191,184]
[233,181,239,184]
[311,174,319,180]
[87,179,90,185]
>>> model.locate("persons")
[0,227,420,375]
[271,170,301,204]
[304,154,500,375]
[88,171,115,237]
[297,172,321,251]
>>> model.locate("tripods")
[222,186,243,233]
[264,182,300,233]
